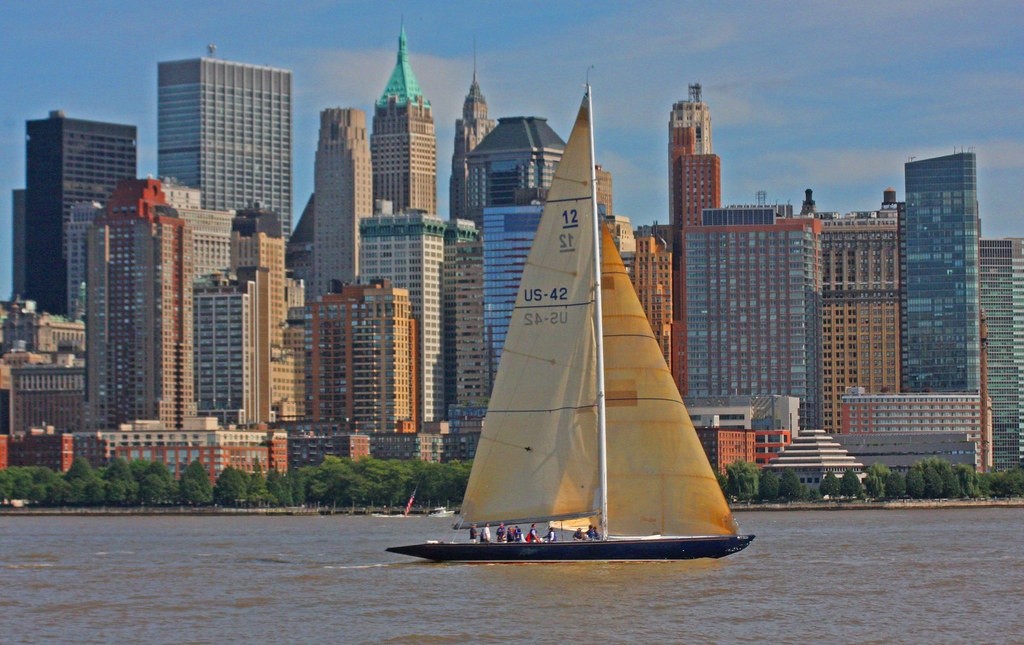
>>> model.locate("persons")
[482,522,600,542]
[469,523,480,543]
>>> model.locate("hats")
[577,528,581,530]
[499,522,504,525]
[471,523,478,526]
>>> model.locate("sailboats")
[385,65,758,564]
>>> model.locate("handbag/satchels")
[525,533,532,542]
